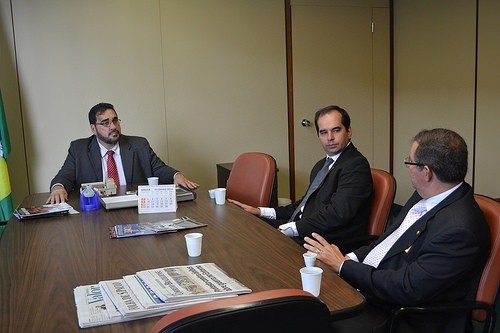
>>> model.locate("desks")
[0,183,368,333]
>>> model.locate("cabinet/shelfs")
[217,162,279,208]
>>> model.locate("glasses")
[402,156,425,168]
[94,119,121,127]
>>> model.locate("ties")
[106,151,119,188]
[362,198,428,270]
[287,157,334,222]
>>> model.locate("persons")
[304,129,491,333]
[227,106,375,257]
[47,103,200,205]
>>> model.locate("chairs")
[150,289,333,333]
[226,152,276,208]
[384,194,500,333]
[324,169,397,254]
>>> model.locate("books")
[109,216,208,239]
[14,203,72,221]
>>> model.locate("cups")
[184,233,203,257]
[208,189,214,199]
[214,188,226,204]
[147,177,159,185]
[303,253,317,267]
[299,267,324,297]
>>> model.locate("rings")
[316,249,320,253]
